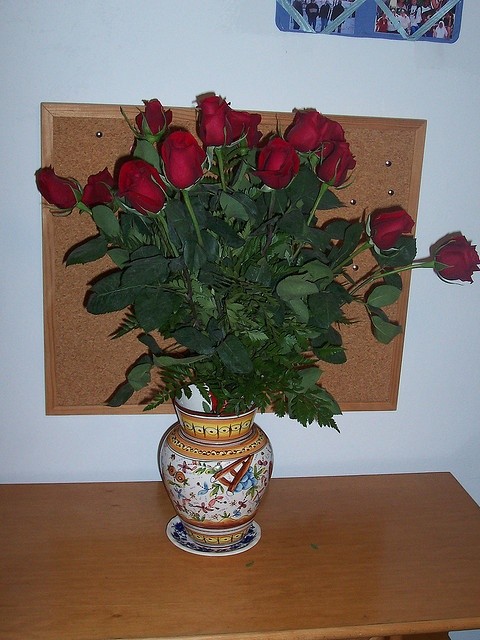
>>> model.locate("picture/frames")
[39,103,426,416]
[276,0,463,43]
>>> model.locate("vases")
[156,381,274,556]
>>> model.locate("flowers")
[34,93,480,432]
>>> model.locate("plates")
[165,515,263,557]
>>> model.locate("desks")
[0,472,479,638]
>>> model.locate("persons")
[322,0,331,29]
[336,0,346,33]
[306,0,320,29]
[375,2,454,39]
